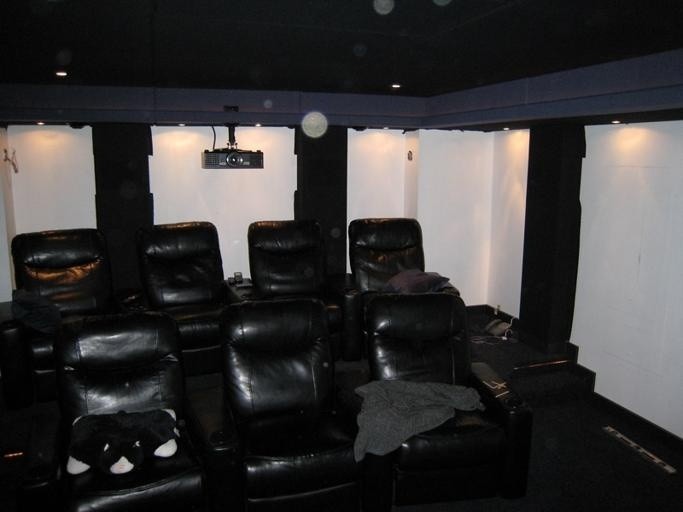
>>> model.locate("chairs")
[0,206,653,511]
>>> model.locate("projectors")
[201,149,264,168]
